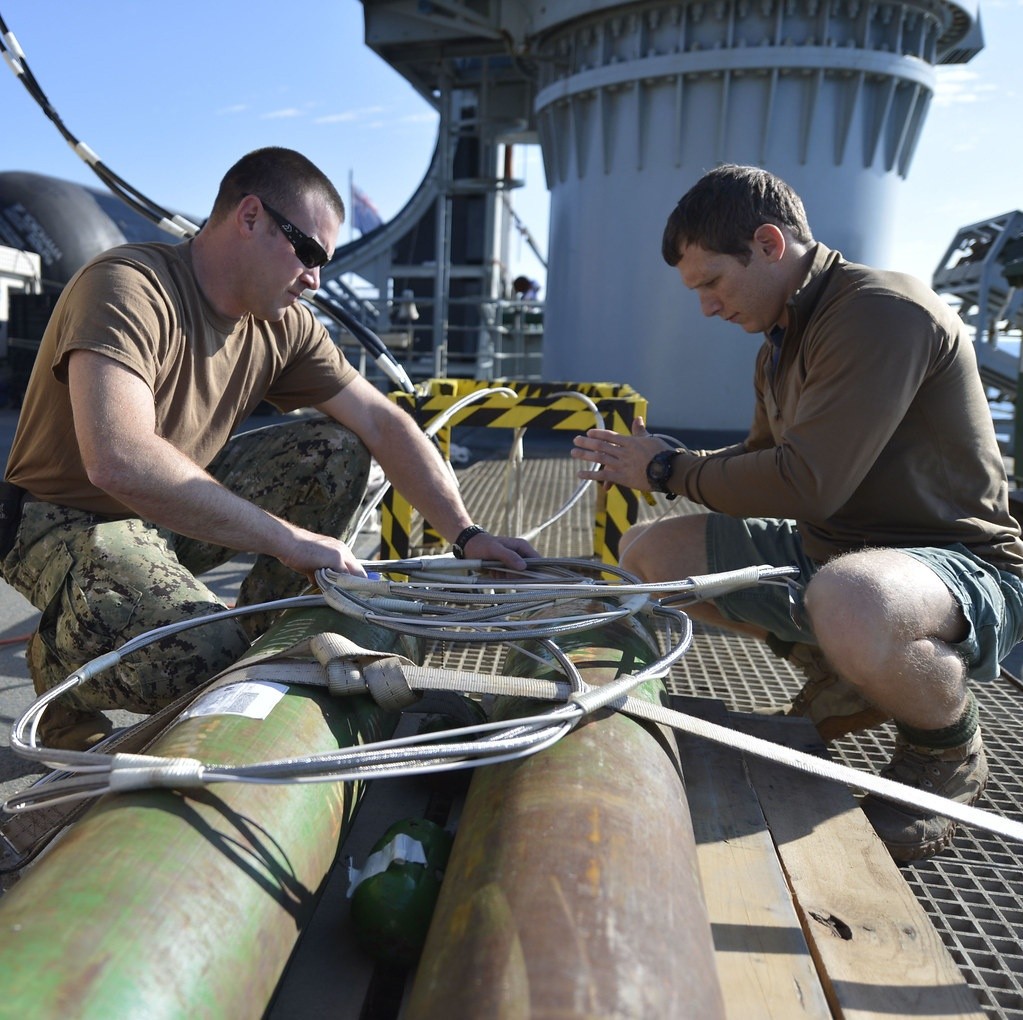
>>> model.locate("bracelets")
[452,525,484,560]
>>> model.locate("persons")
[571,166,1023,861]
[0,148,537,752]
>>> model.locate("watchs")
[646,449,684,500]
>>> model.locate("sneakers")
[860,726,990,859]
[777,644,893,750]
[24,630,111,752]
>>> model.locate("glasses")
[238,191,331,271]
